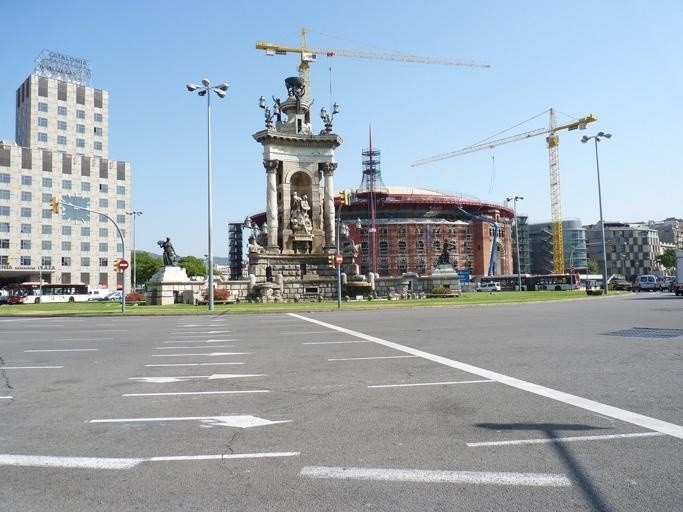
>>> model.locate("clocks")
[61,193,91,225]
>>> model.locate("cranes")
[410,108,599,274]
[255,27,492,124]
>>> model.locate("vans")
[632,275,656,292]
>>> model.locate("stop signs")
[119,260,129,270]
[336,254,344,264]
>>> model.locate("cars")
[608,279,632,291]
[88,289,144,305]
[586,279,604,295]
[476,282,500,292]
[0,295,7,304]
[658,276,683,296]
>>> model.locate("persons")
[272,96,280,121]
[292,89,304,113]
[159,237,177,267]
[290,191,312,236]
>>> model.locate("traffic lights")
[339,188,351,206]
[49,196,60,213]
[113,260,118,272]
[328,255,335,269]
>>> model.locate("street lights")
[125,211,143,293]
[506,196,524,291]
[569,246,575,290]
[581,131,611,294]
[186,79,230,310]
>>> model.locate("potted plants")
[125,293,146,308]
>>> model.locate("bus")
[7,282,88,305]
[476,273,580,291]
